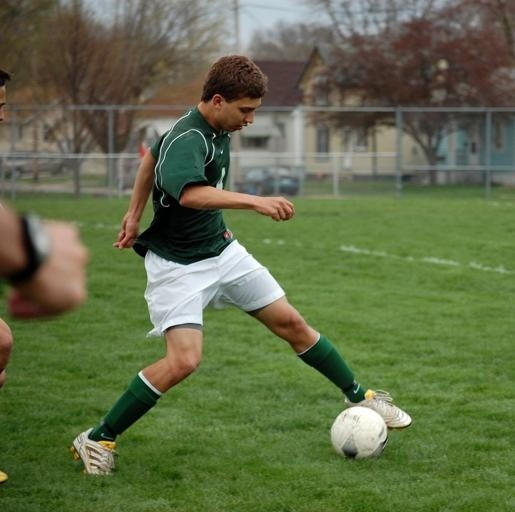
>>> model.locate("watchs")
[3,209,51,288]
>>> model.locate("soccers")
[331,405,389,460]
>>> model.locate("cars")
[234,165,300,197]
[1,148,74,180]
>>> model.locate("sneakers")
[344,389,412,429]
[70,427,116,476]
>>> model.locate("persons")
[0,192,93,487]
[67,52,415,480]
[0,63,15,126]
